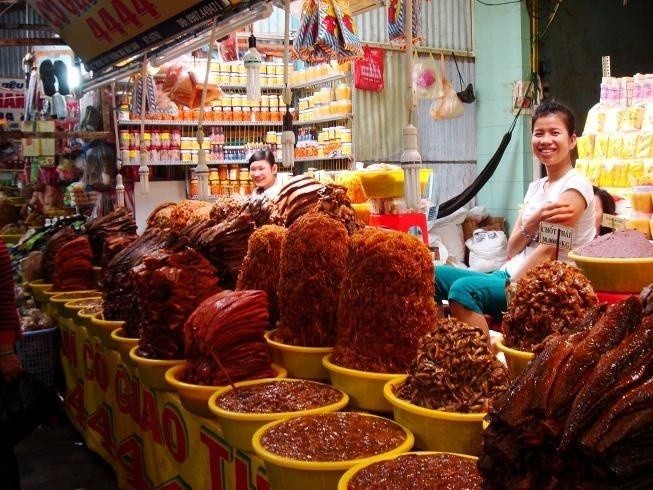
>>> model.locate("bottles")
[117,55,359,206]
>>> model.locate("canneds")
[190,166,256,198]
[267,129,281,143]
[294,58,349,87]
[313,126,353,156]
[299,84,353,120]
[197,58,293,87]
[180,93,295,122]
[120,130,214,162]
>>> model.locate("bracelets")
[519,226,538,241]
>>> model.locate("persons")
[246,150,281,198]
[589,185,614,240]
[436,101,595,346]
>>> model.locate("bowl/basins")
[0,168,652,490]
[564,240,653,296]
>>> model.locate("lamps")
[82,0,277,103]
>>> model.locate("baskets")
[17,325,59,385]
